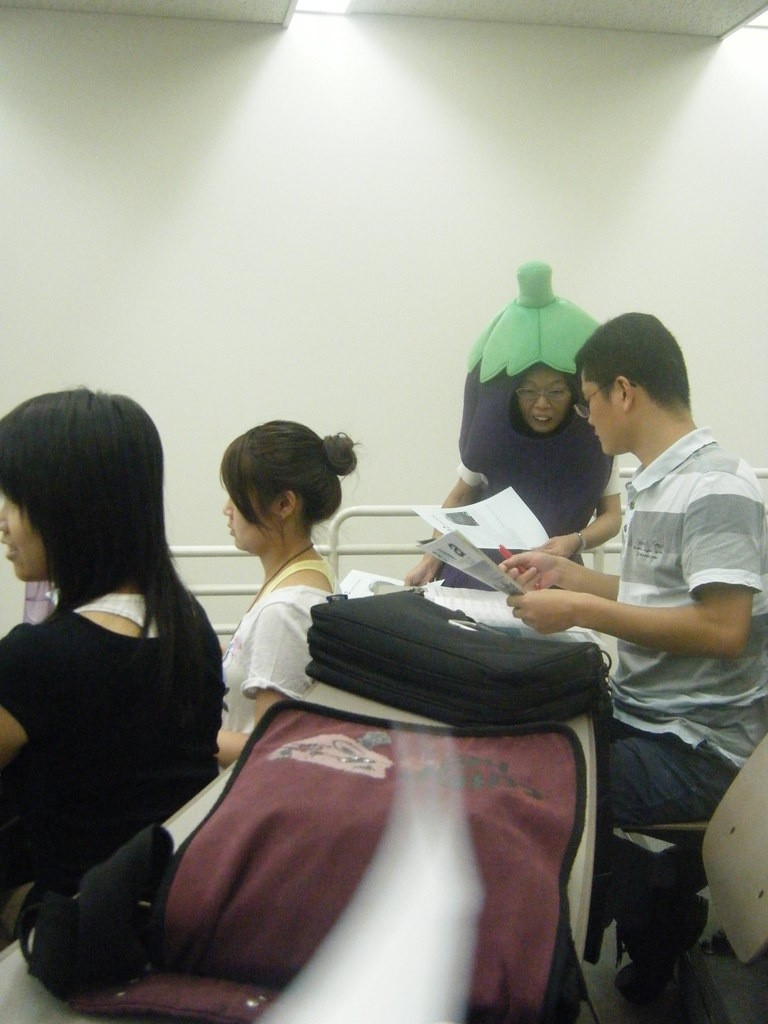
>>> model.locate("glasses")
[514,387,572,403]
[574,380,637,419]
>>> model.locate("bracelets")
[576,531,586,556]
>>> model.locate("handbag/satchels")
[305,589,614,747]
[21,701,587,1024]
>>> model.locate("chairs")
[621,821,708,832]
[702,730,768,967]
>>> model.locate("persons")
[213,420,361,775]
[404,367,623,593]
[497,313,768,1005]
[0,387,223,951]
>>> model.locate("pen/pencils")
[497,545,540,588]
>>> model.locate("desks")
[0,682,598,1024]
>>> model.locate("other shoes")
[613,893,707,1005]
